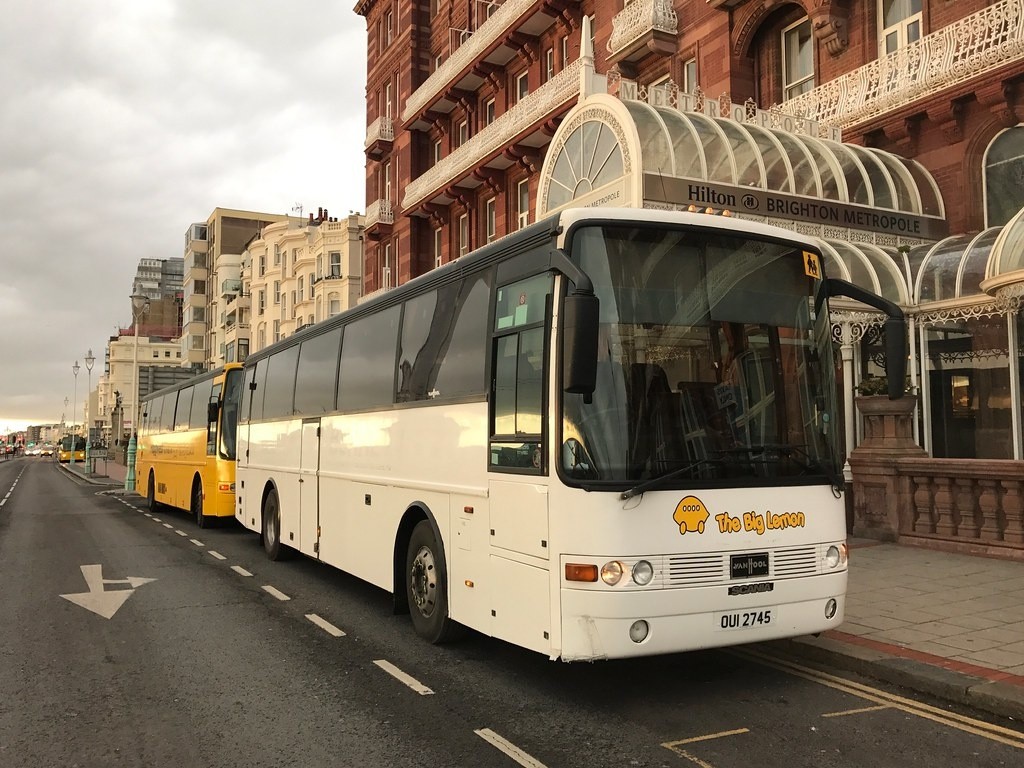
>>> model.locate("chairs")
[605,360,731,457]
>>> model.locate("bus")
[229,205,912,668]
[58,434,86,462]
[133,360,244,529]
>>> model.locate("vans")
[40,445,54,457]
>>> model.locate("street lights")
[122,283,154,490]
[68,360,81,465]
[83,347,96,474]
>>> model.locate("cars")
[24,446,41,456]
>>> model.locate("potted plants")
[852,376,920,416]
